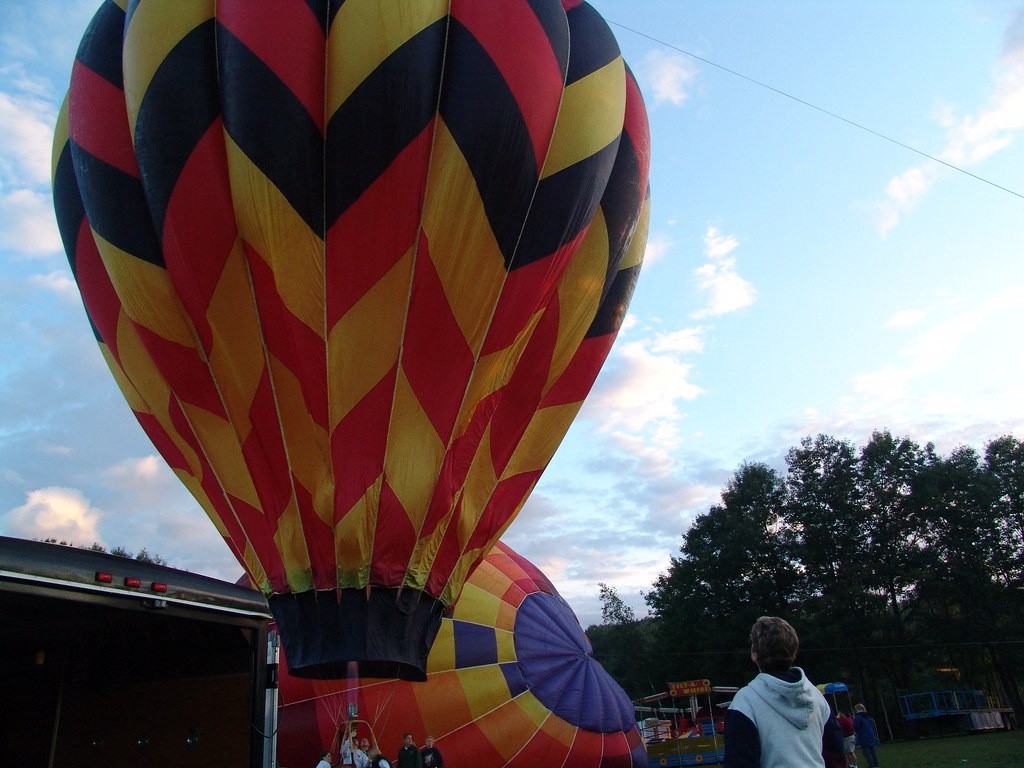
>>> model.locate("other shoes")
[849,763,858,768]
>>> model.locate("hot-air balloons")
[233,538,653,766]
[50,0,652,768]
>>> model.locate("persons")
[724,616,847,768]
[316,730,444,768]
[837,703,880,768]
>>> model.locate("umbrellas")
[816,678,849,714]
